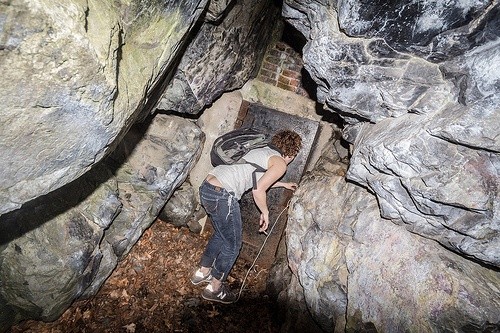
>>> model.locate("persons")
[191,130,306,306]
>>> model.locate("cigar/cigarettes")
[260,228,268,235]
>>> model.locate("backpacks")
[210,126,285,172]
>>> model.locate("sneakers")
[200,282,239,304]
[190,267,213,286]
